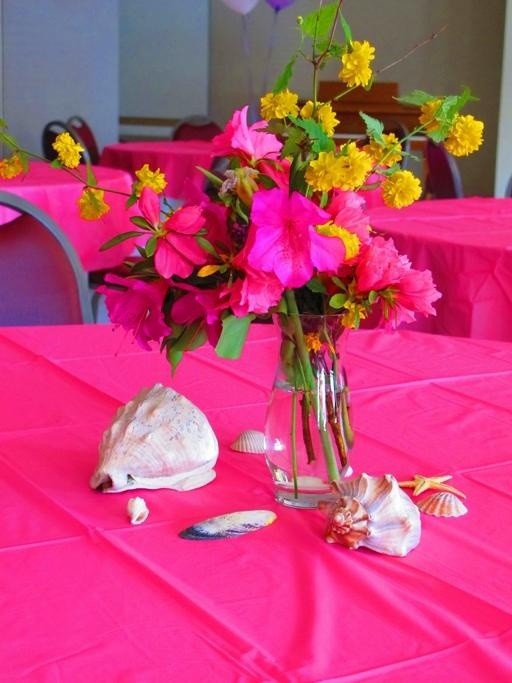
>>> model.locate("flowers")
[0,0,486,499]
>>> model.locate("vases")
[263,314,353,508]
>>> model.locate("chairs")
[43,120,92,164]
[0,191,112,326]
[66,115,99,165]
